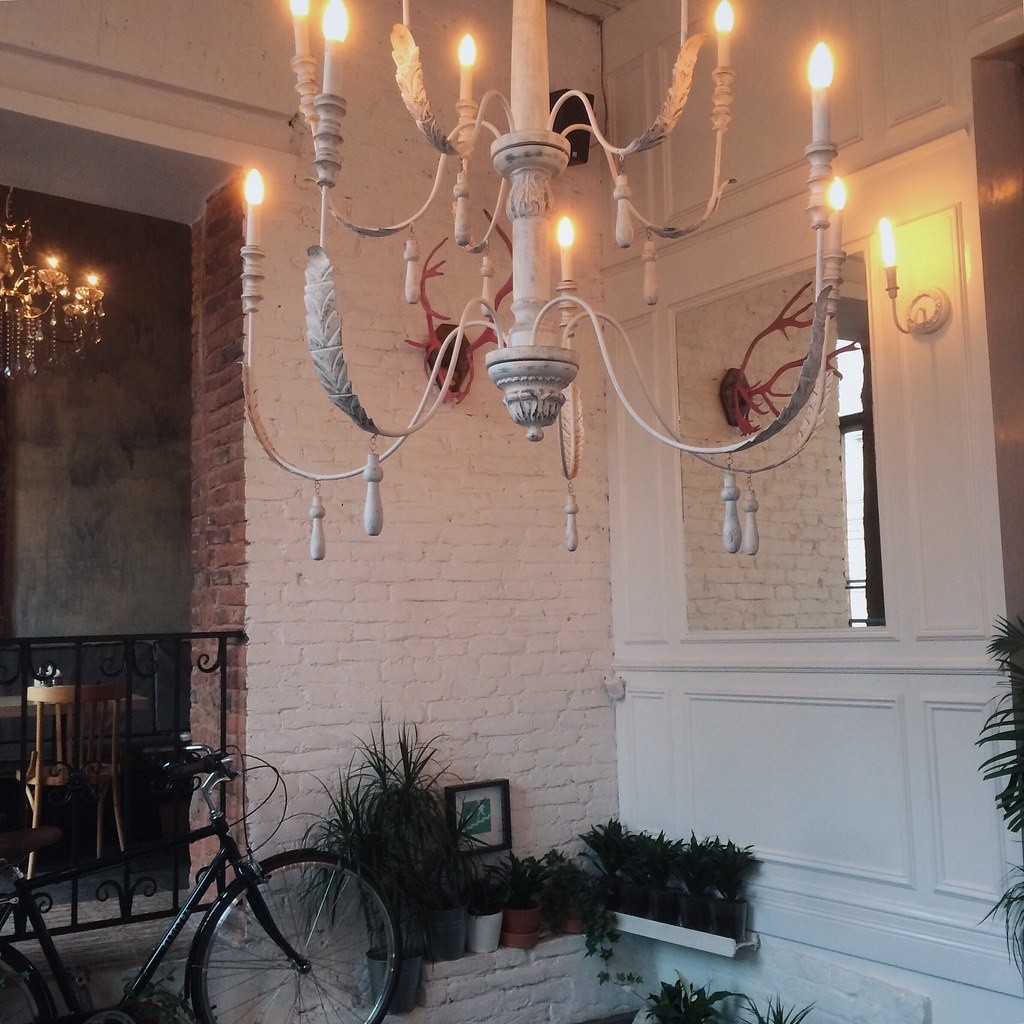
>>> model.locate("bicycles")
[0,733,401,1024]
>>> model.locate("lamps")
[239,1,847,561]
[876,217,950,336]
[0,184,107,383]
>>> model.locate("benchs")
[0,639,189,775]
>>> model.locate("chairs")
[14,683,125,881]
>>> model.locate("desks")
[0,694,151,719]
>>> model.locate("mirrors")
[672,249,888,634]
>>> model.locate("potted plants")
[424,897,467,961]
[545,820,762,1024]
[477,846,555,949]
[462,874,504,954]
[284,693,496,1016]
